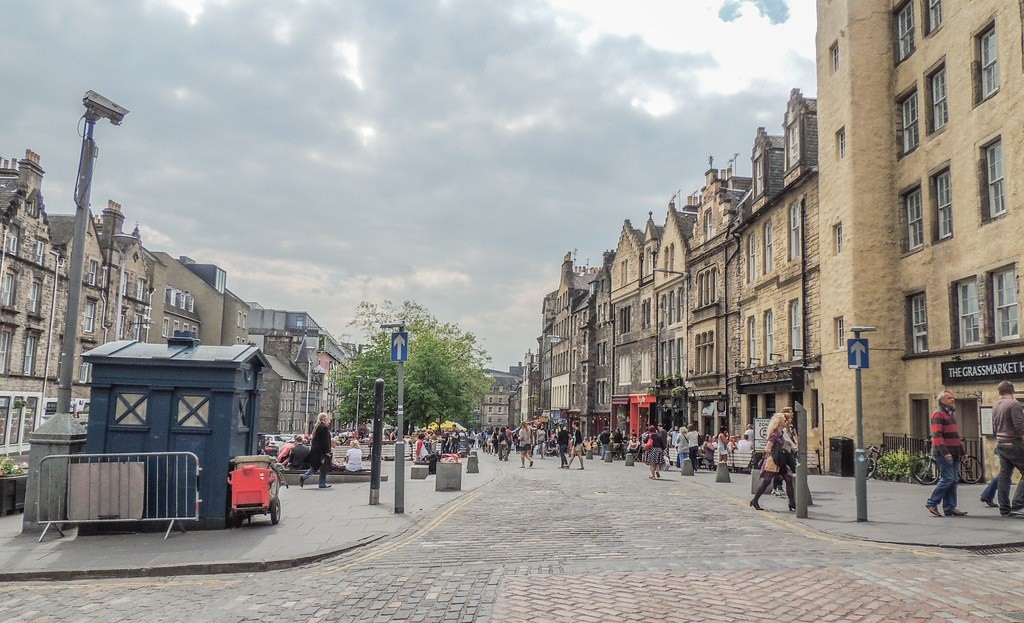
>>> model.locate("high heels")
[788,503,796,511]
[750,497,763,510]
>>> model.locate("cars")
[259,434,294,457]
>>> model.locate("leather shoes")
[981,496,998,507]
[926,503,942,516]
[946,508,968,516]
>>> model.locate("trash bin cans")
[829,435,855,477]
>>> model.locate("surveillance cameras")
[82,90,130,126]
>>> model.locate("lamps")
[547,335,568,340]
[742,363,770,376]
[654,267,689,279]
[773,358,783,370]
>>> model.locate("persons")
[300,412,332,489]
[462,420,753,479]
[981,381,1024,518]
[750,407,796,512]
[441,424,461,455]
[925,391,968,517]
[277,427,441,474]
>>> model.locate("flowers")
[0,457,25,476]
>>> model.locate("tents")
[365,419,467,433]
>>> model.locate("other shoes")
[519,465,525,468]
[319,484,332,488]
[529,461,534,467]
[649,475,655,479]
[656,471,660,477]
[299,474,305,487]
[1001,507,1024,516]
[772,489,783,496]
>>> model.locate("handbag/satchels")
[644,438,653,450]
[698,434,703,447]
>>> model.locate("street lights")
[355,375,363,430]
[380,323,406,513]
[305,346,315,433]
[849,326,876,522]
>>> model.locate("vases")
[0,475,27,517]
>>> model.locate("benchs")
[413,460,430,465]
[411,466,429,479]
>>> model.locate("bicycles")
[912,434,982,485]
[865,444,886,481]
